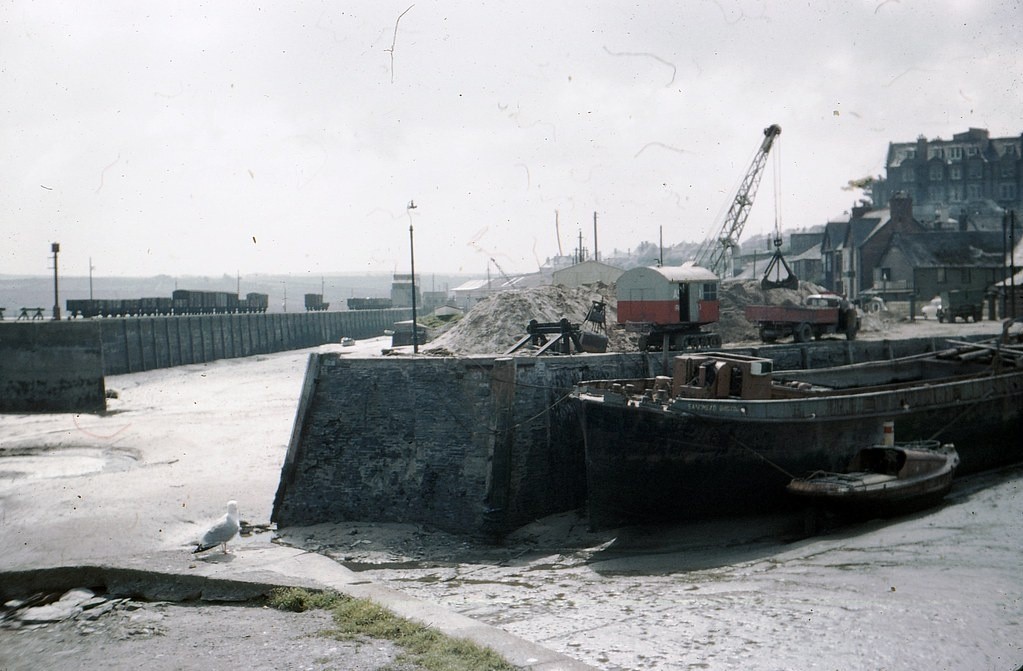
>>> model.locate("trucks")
[744,293,863,345]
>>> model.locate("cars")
[921,297,942,320]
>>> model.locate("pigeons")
[191,500,240,554]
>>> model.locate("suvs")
[936,287,985,325]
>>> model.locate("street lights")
[407,199,418,353]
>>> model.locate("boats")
[785,440,961,531]
[567,312,1023,536]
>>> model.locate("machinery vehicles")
[616,124,782,353]
